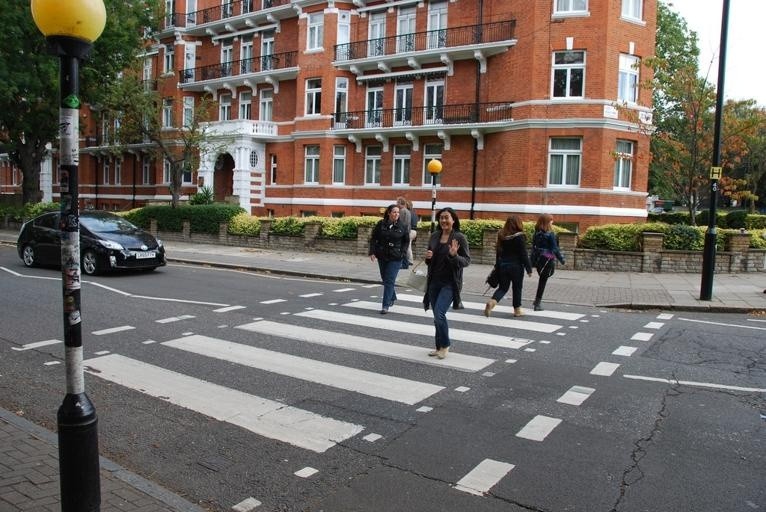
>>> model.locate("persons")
[397,196,411,269]
[484,215,533,317]
[530,213,566,311]
[368,205,410,314]
[423,207,472,358]
[406,200,418,265]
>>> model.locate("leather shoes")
[382,306,389,313]
[389,296,396,306]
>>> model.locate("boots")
[485,299,497,317]
[533,297,544,311]
[514,307,524,317]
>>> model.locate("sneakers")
[429,346,449,358]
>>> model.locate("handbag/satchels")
[488,269,499,288]
[530,250,537,267]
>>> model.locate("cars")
[17,210,166,275]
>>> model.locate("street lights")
[30,0,108,512]
[428,159,442,240]
